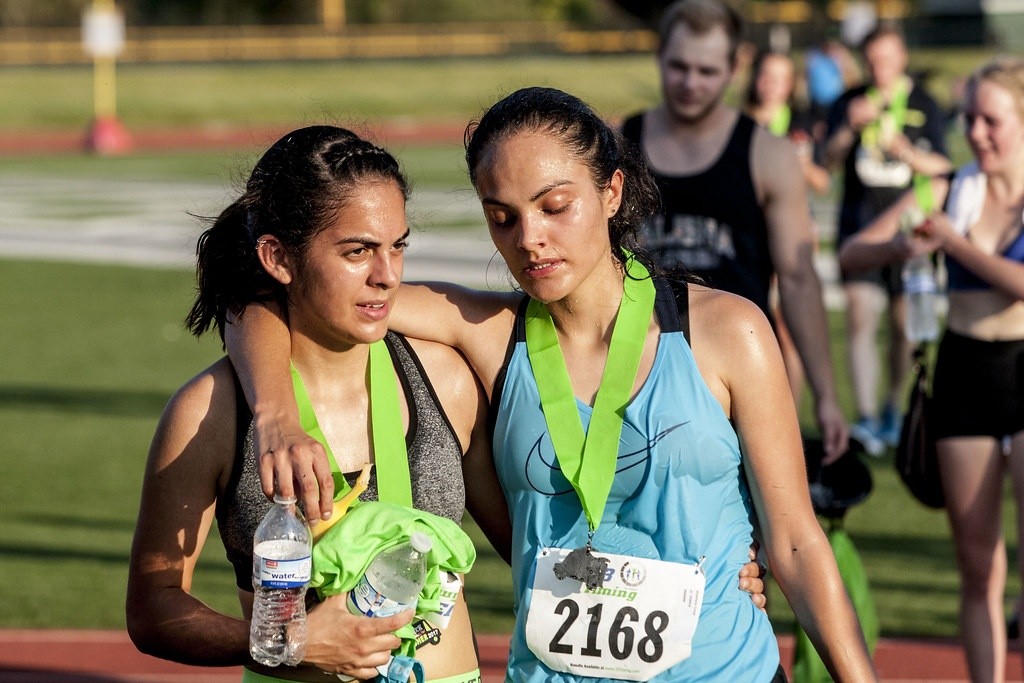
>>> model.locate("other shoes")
[854,404,901,448]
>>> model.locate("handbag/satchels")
[894,368,950,508]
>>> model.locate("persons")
[839,59,1024,683]
[125,125,766,683]
[225,86,879,683]
[606,0,848,464]
[744,27,958,460]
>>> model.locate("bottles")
[324,533,432,680]
[249,495,313,666]
[902,258,939,338]
